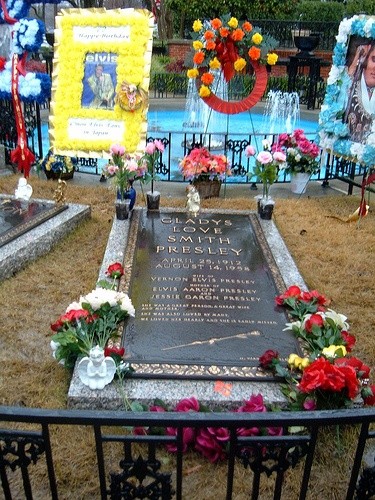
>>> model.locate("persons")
[88,64,114,109]
[340,43,375,143]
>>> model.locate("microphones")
[359,49,365,56]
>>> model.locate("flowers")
[104,345,150,437]
[129,395,307,463]
[41,148,74,174]
[313,15,375,167]
[97,263,124,291]
[52,11,149,155]
[0,0,51,104]
[245,129,322,200]
[259,286,375,412]
[179,147,232,184]
[103,139,163,201]
[186,18,279,98]
[49,287,135,371]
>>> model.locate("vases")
[192,180,223,199]
[146,191,161,212]
[115,199,131,220]
[45,169,75,181]
[255,195,275,220]
[117,180,136,210]
[290,172,309,194]
[293,36,322,57]
